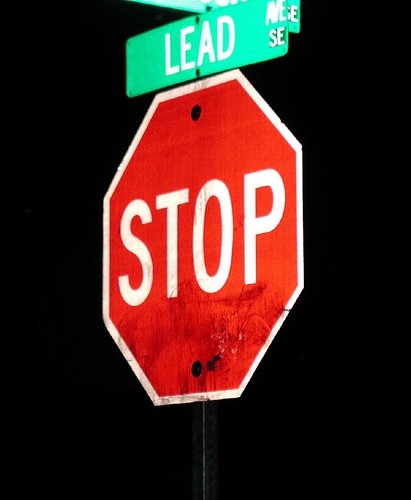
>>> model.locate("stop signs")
[103,68,305,406]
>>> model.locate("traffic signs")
[122,1,291,98]
[127,0,302,37]
